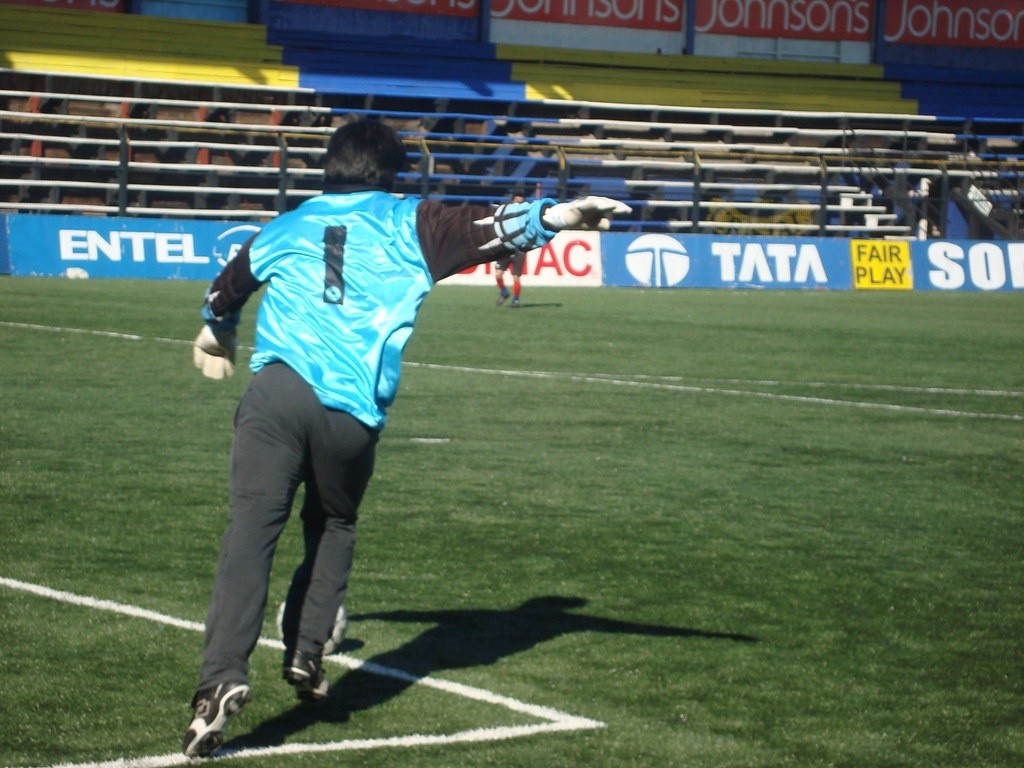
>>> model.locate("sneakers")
[183,680,250,756]
[283,650,344,706]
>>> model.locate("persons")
[182,119,632,756]
[496,194,528,306]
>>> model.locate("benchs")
[0,0,1024,240]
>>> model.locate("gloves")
[193,325,235,379]
[542,195,632,231]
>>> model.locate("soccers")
[277,599,347,657]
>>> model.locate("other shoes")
[496,293,510,304]
[512,299,519,306]
